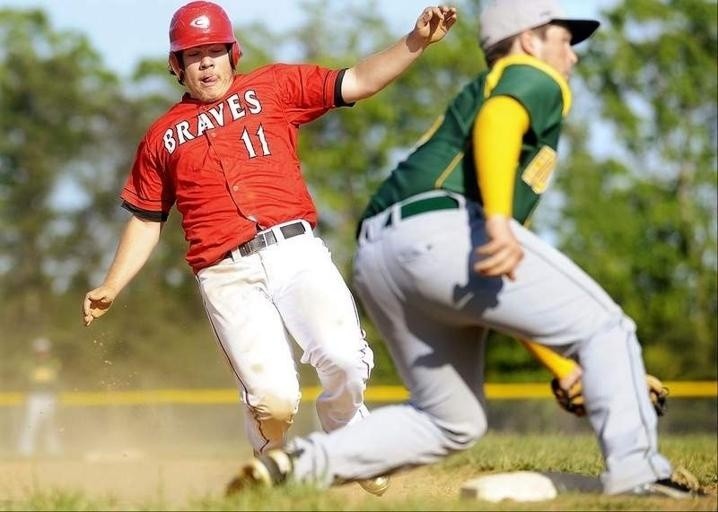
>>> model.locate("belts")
[223,221,309,262]
[364,194,457,239]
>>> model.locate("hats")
[477,0,602,53]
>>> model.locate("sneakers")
[603,460,707,500]
[222,446,293,499]
[359,475,397,497]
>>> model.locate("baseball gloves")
[552,369,666,416]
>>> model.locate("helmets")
[168,0,242,72]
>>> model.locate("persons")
[84,2,459,495]
[223,1,708,499]
